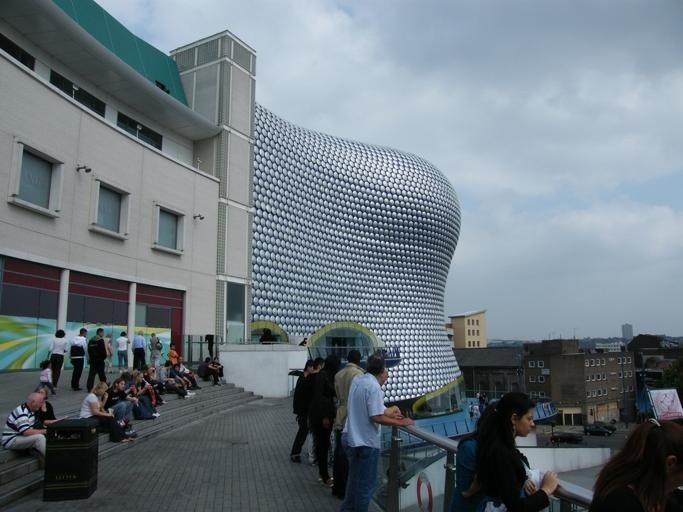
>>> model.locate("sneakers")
[318,476,333,487]
[292,455,302,462]
[196,385,201,389]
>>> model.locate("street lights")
[551,422,555,442]
[638,369,647,387]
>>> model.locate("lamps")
[194,213,204,221]
[77,166,92,173]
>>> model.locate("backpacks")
[478,497,507,512]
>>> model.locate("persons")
[289,349,415,512]
[448,400,499,512]
[470,391,489,423]
[476,391,560,512]
[588,419,683,511]
[0,328,226,467]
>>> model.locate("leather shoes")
[74,388,83,391]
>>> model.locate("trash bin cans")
[42,417,99,502]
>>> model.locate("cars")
[549,432,584,444]
[584,420,617,437]
